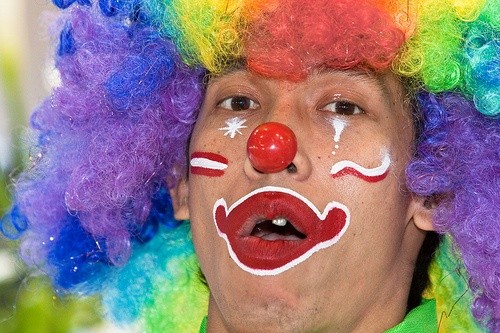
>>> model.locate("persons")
[15,0,497,333]
